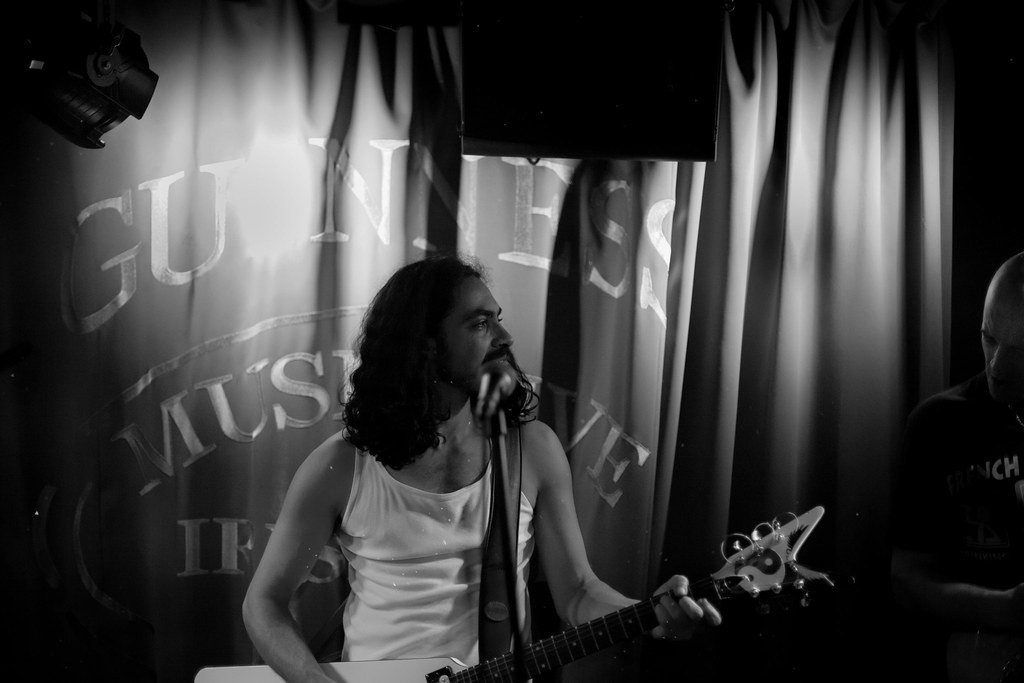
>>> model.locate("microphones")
[474,362,517,420]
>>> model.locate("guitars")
[193,503,838,683]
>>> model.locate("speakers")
[460,4,722,160]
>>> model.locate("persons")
[237,259,726,682]
[856,245,1024,682]
